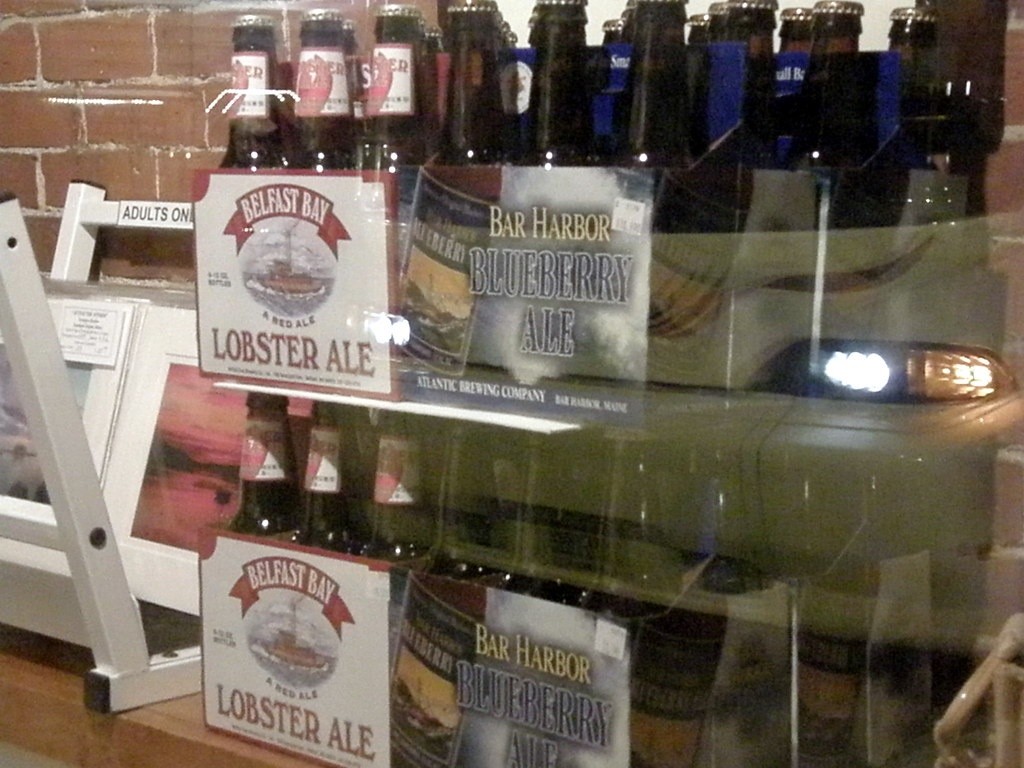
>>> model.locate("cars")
[329,134,1023,667]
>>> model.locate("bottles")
[629,605,730,768]
[222,0,986,207]
[389,573,486,767]
[232,391,943,666]
[399,169,503,388]
